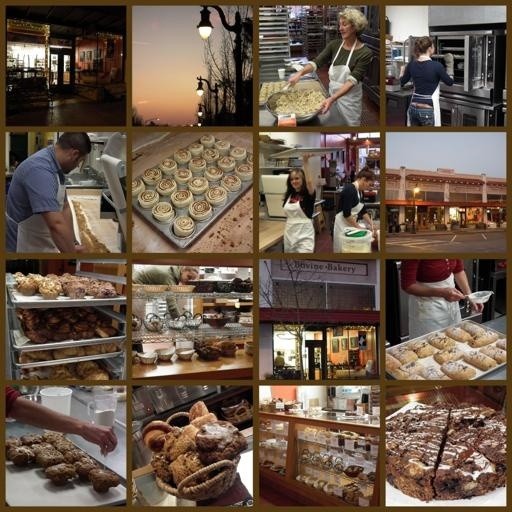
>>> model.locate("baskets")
[142,285,168,293]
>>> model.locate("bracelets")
[71,239,80,245]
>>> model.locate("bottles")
[386,70,393,85]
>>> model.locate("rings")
[105,438,109,444]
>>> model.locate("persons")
[399,259,485,341]
[399,36,454,127]
[329,167,377,254]
[5,383,118,458]
[5,132,91,256]
[288,7,373,128]
[274,351,285,370]
[281,151,317,254]
[131,264,199,324]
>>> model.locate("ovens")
[403,28,499,127]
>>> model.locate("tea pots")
[89,393,118,424]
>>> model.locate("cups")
[277,68,286,80]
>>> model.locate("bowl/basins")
[155,348,174,360]
[176,347,195,359]
[137,351,157,364]
[132,311,202,331]
[203,314,231,329]
[265,90,326,122]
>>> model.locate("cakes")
[296,427,378,503]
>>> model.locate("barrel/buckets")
[337,229,376,254]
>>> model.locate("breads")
[131,134,253,236]
[141,401,248,488]
[12,272,117,390]
[385,322,507,381]
[5,432,120,493]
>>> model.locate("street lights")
[198,117,206,127]
[198,103,211,127]
[196,76,218,127]
[196,5,244,126]
[410,183,420,234]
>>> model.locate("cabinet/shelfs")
[257,408,380,507]
[130,291,254,350]
[5,272,128,387]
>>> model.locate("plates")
[5,431,126,507]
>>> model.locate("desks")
[258,218,286,253]
